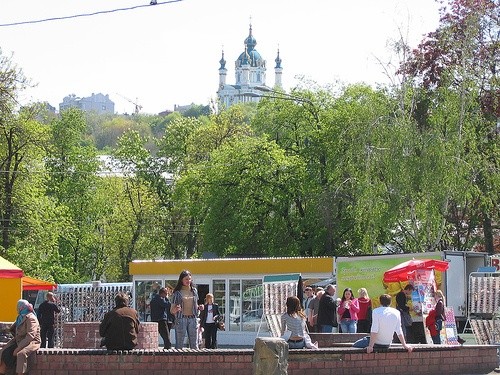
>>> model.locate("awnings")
[23,276,58,291]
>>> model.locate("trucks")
[324,250,489,344]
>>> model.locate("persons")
[99,293,141,351]
[357,288,372,333]
[170,270,205,350]
[0,299,42,375]
[29,304,36,315]
[337,287,359,333]
[145,281,175,349]
[196,293,222,349]
[396,284,415,343]
[426,289,445,344]
[37,291,61,348]
[279,296,318,349]
[301,282,340,333]
[353,294,413,354]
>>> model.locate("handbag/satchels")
[217,322,225,331]
[428,316,442,330]
[402,312,412,326]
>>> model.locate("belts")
[182,314,195,319]
[288,338,303,342]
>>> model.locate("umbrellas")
[382,256,449,306]
